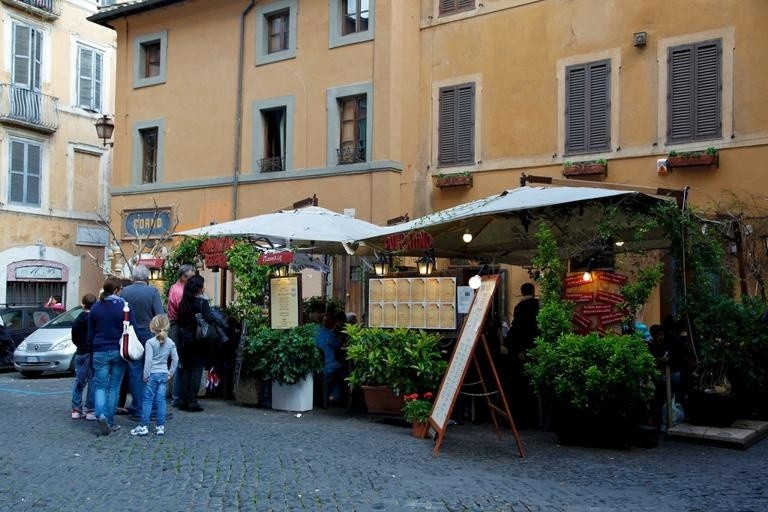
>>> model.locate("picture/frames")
[267,273,302,330]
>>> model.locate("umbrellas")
[165,203,383,277]
[354,185,674,282]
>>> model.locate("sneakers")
[72,400,204,435]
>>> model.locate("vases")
[411,419,424,437]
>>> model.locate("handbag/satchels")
[196,313,219,342]
[119,325,144,361]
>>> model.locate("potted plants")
[665,147,720,169]
[562,159,609,178]
[674,272,765,427]
[250,322,321,413]
[434,170,473,189]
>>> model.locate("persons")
[119,264,173,422]
[306,299,359,406]
[115,277,141,413]
[650,324,660,333]
[176,274,221,411]
[503,282,540,353]
[71,293,100,421]
[130,314,181,436]
[223,316,239,400]
[87,277,145,436]
[166,263,207,399]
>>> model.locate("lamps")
[371,249,395,277]
[462,229,473,243]
[336,315,449,418]
[93,114,115,147]
[414,248,435,275]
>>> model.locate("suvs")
[0,305,65,369]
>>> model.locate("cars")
[12,305,88,377]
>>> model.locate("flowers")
[401,389,434,423]
